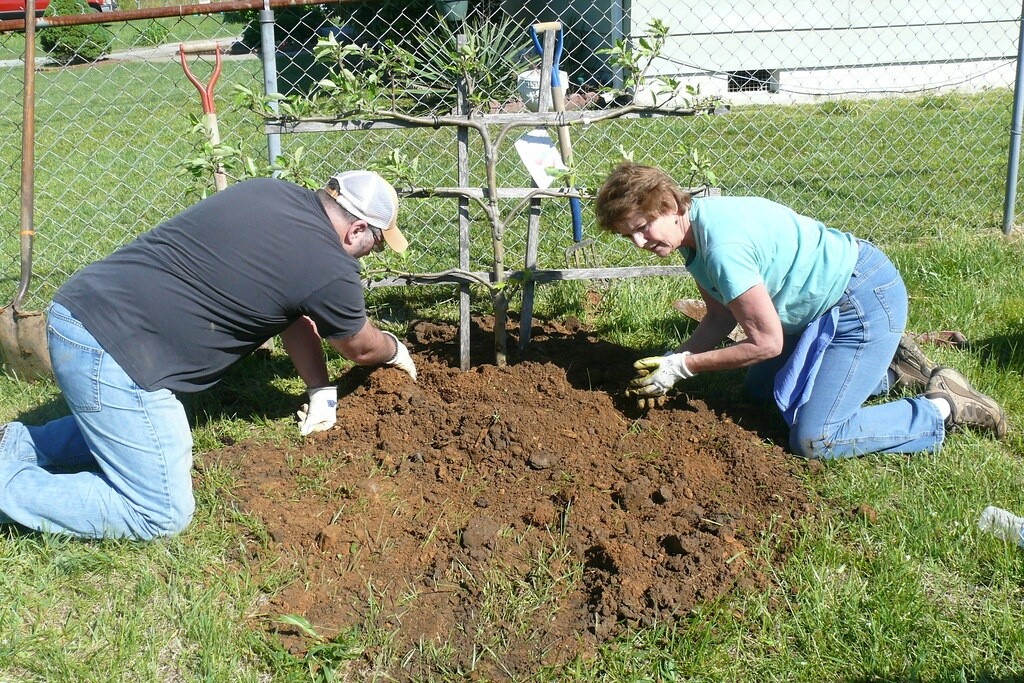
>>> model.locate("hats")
[324,170,409,254]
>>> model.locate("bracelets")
[383,331,398,362]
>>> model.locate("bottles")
[978,505,1024,548]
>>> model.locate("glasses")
[368,225,385,252]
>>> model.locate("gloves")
[297,385,338,437]
[380,330,417,383]
[624,349,700,409]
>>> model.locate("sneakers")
[889,338,936,388]
[925,366,1007,439]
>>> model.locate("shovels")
[674,297,966,351]
[1,0,55,382]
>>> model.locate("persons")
[596,165,1009,460]
[0,170,418,542]
